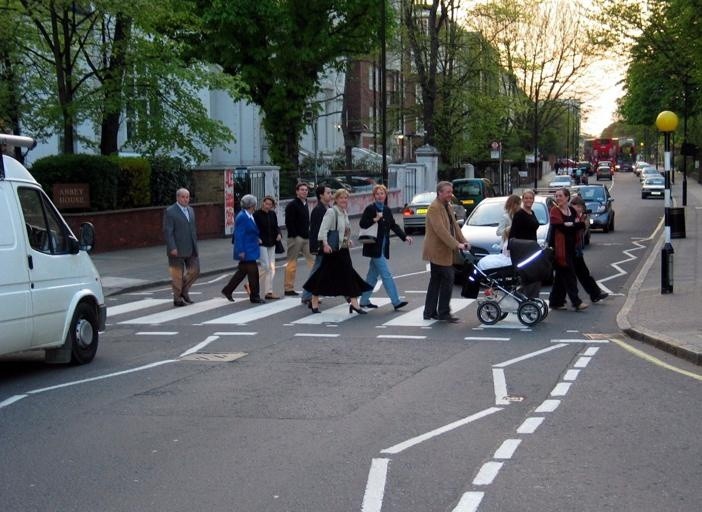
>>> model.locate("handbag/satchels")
[317,229,339,252]
[275,241,284,254]
[358,223,379,244]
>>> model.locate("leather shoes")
[394,302,408,310]
[284,290,299,295]
[221,288,234,301]
[174,292,193,306]
[424,313,460,322]
[244,282,280,302]
[350,303,378,314]
[302,297,321,313]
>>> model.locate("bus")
[583,138,637,165]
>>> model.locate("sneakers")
[592,292,608,302]
[550,303,589,311]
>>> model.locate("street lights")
[655,110,678,241]
[675,87,699,205]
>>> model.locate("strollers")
[458,244,548,326]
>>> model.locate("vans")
[0,132,107,368]
[453,177,498,213]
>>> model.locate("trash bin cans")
[668,207,686,239]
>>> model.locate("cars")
[578,160,615,180]
[549,175,576,193]
[571,182,615,233]
[615,159,665,199]
[403,192,469,234]
[453,197,558,287]
[560,159,576,166]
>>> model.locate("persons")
[284,182,315,295]
[301,185,373,315]
[244,196,282,299]
[496,191,539,301]
[549,189,608,310]
[164,188,200,306]
[422,181,471,321]
[359,185,413,310]
[221,194,265,303]
